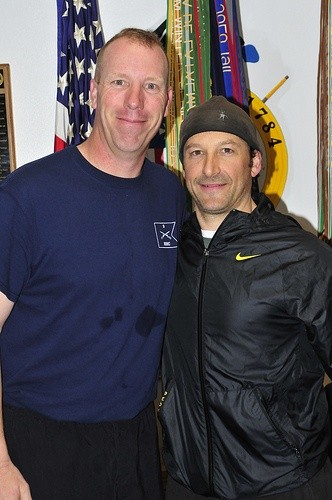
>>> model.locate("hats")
[178,95,262,164]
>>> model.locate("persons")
[0,29,190,500]
[155,94,331,500]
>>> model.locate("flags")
[52,0,104,153]
